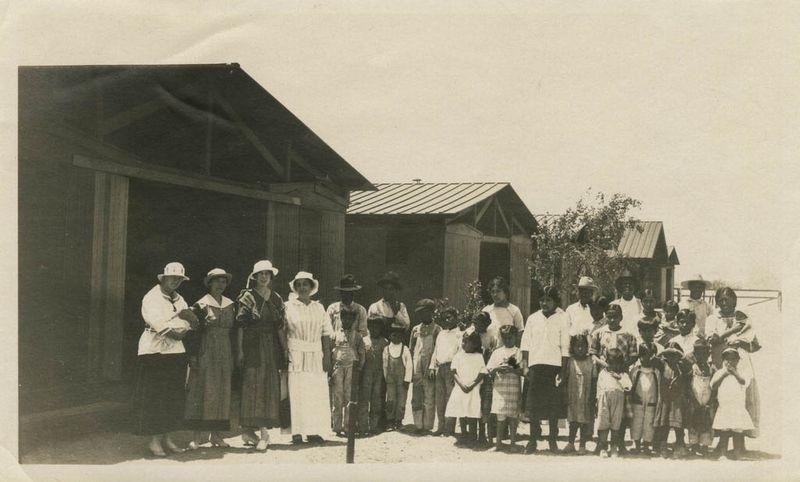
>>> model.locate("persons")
[327,275,763,462]
[182,267,238,452]
[234,259,289,452]
[134,261,198,459]
[282,270,336,446]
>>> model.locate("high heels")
[242,432,270,451]
[209,435,229,449]
[189,437,201,451]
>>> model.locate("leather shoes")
[150,434,184,457]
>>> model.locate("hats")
[376,271,402,291]
[415,299,434,313]
[681,273,711,287]
[157,262,190,281]
[333,274,362,292]
[204,268,232,288]
[289,271,318,296]
[615,271,640,292]
[573,277,599,292]
[249,260,279,280]
